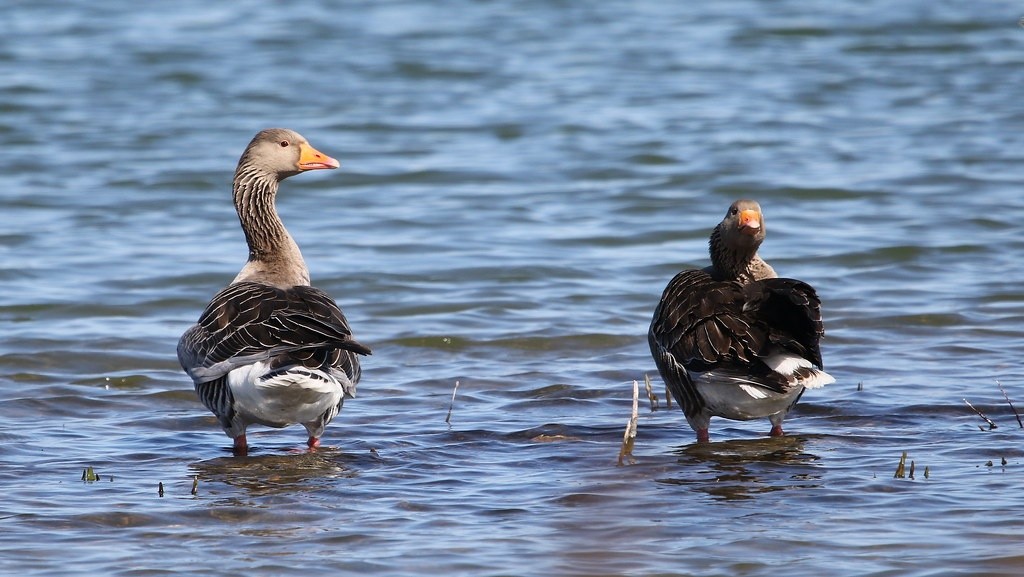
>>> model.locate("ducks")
[648,199,836,443]
[176,128,373,454]
